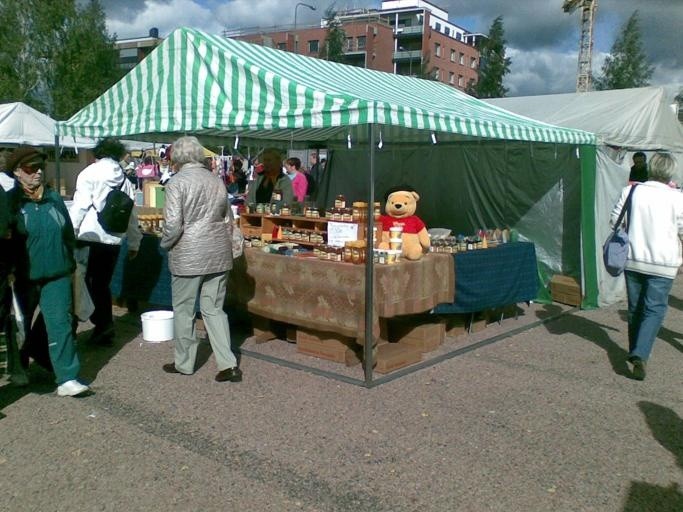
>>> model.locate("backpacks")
[89,172,133,234]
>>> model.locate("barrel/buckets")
[141,310,174,342]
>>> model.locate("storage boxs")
[550,274,581,306]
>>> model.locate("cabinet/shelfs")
[239,212,384,249]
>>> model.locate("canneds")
[313,245,342,261]
[342,209,352,221]
[311,208,319,219]
[335,195,346,210]
[333,209,341,221]
[281,228,326,244]
[325,209,333,221]
[306,207,312,218]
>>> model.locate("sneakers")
[90,332,114,347]
[57,380,88,397]
[13,369,31,386]
[632,356,647,379]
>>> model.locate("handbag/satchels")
[225,201,244,258]
[73,270,95,322]
[603,230,629,276]
[136,165,158,178]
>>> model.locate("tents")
[304,78,682,310]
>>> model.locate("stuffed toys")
[375,190,431,261]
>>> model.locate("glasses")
[20,163,45,174]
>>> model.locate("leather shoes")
[215,367,241,382]
[163,362,178,373]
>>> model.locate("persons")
[609,151,683,377]
[156,134,243,383]
[109,147,325,212]
[70,136,144,344]
[0,145,26,352]
[627,151,649,182]
[0,144,89,397]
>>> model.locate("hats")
[124,165,135,170]
[5,147,48,178]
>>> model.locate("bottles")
[233,188,404,266]
[136,212,166,233]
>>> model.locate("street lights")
[291,1,315,51]
